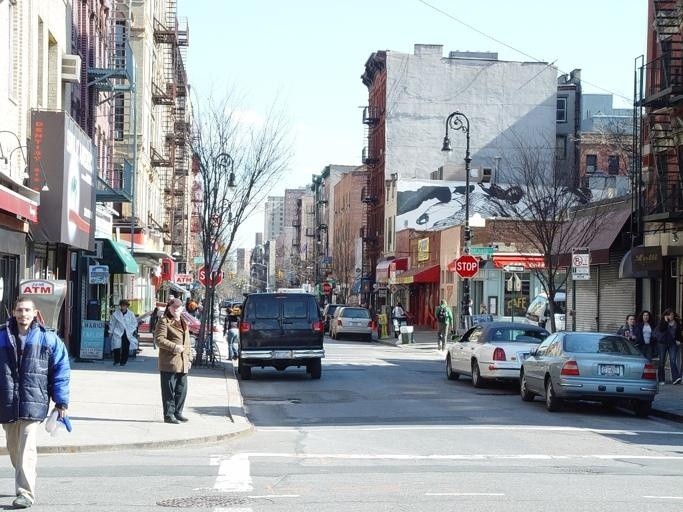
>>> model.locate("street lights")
[213,196,234,236]
[243,261,270,292]
[204,152,238,362]
[441,111,475,328]
[315,222,330,273]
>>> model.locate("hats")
[169,298,182,307]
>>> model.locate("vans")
[523,291,567,331]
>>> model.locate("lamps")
[7,141,49,195]
[0,129,33,183]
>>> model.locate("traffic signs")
[502,263,523,274]
[470,247,493,255]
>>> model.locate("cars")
[444,320,551,388]
[516,330,659,417]
[328,306,374,341]
[216,296,244,332]
[132,302,214,345]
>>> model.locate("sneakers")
[672,378,681,385]
[658,382,664,386]
[13,495,32,508]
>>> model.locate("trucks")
[277,286,307,294]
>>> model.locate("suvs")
[320,303,348,332]
[235,293,326,378]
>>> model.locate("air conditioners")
[61,53,82,84]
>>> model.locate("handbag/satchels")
[654,326,666,342]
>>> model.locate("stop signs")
[197,264,222,289]
[318,283,331,295]
[454,255,478,279]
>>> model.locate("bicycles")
[186,330,222,367]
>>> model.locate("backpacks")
[436,305,448,322]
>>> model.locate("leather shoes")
[177,416,188,421]
[172,419,181,424]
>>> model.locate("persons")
[616,314,642,348]
[222,308,239,362]
[186,294,204,319]
[359,299,405,321]
[652,308,683,386]
[636,310,656,362]
[0,296,70,509]
[107,299,138,367]
[481,305,489,315]
[150,307,161,350]
[154,298,192,425]
[434,298,454,352]
